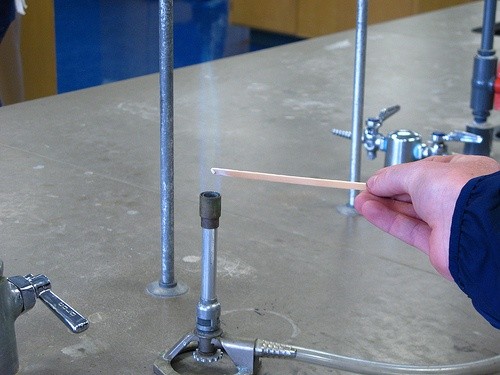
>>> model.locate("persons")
[354,153,500,331]
